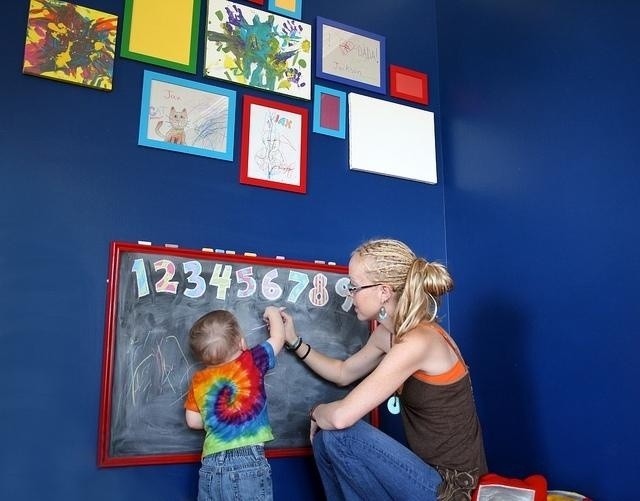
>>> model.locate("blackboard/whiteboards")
[96,240,379,469]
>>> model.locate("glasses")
[345,282,384,297]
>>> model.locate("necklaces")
[387,330,402,419]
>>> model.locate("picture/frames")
[316,15,387,95]
[240,95,308,193]
[267,0,302,21]
[204,0,311,100]
[389,65,428,106]
[138,69,237,162]
[348,92,437,185]
[119,0,201,74]
[313,84,347,140]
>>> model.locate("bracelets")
[307,402,322,422]
[286,334,302,353]
[297,343,312,360]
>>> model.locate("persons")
[276,237,492,501]
[181,304,286,501]
